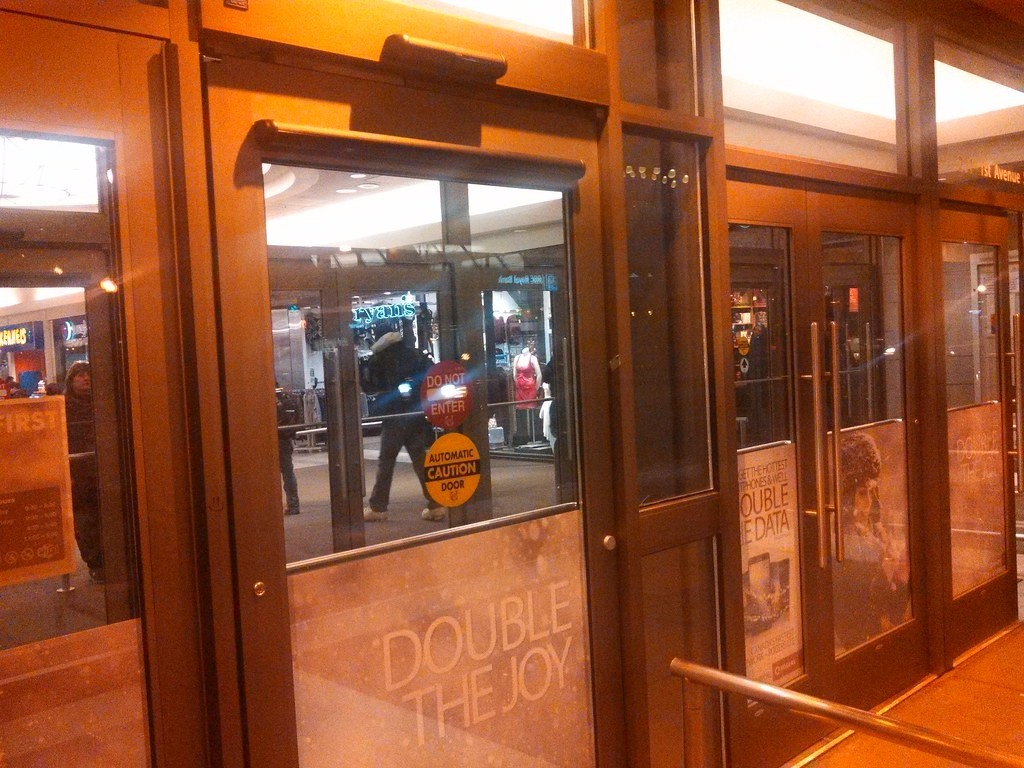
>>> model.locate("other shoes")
[285,508,299,514]
[88,568,103,584]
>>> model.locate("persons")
[5,376,28,398]
[60,359,108,584]
[512,347,541,411]
[44,384,61,396]
[274,382,302,517]
[361,323,446,523]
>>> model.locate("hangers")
[274,386,325,401]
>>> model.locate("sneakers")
[421,507,447,521]
[363,507,388,522]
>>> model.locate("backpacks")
[380,348,424,405]
[277,393,300,438]
